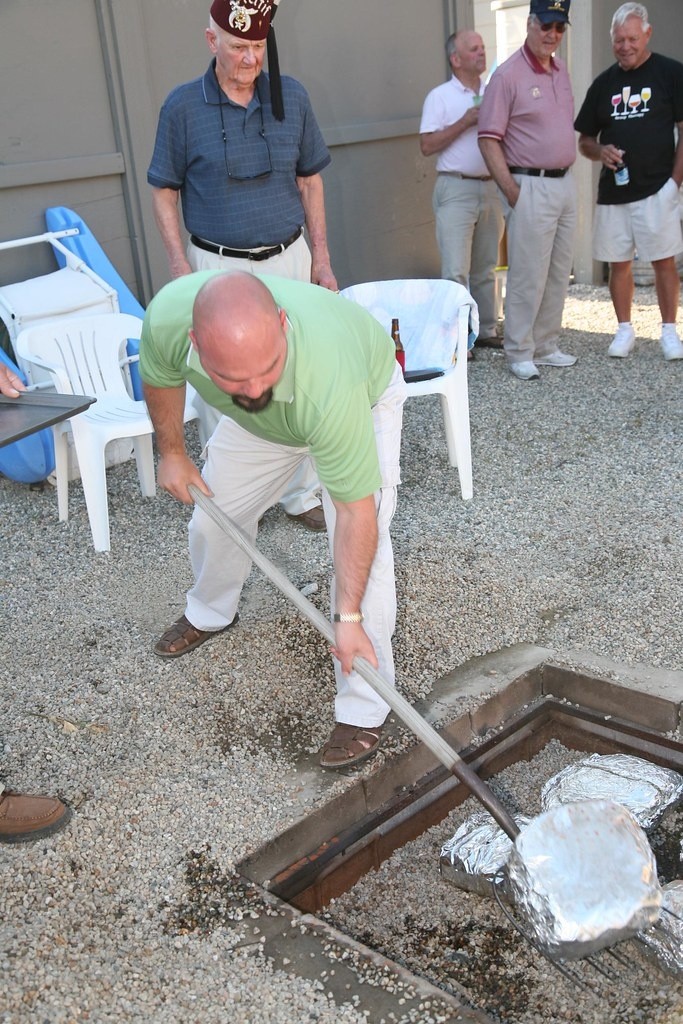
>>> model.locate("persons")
[418,31,504,360]
[0,360,73,842]
[145,0,341,530]
[477,0,579,380]
[138,270,406,769]
[573,2,683,362]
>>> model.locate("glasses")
[532,18,566,33]
[222,132,273,180]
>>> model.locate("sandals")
[319,724,384,768]
[152,612,240,657]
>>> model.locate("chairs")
[341,278,473,503]
[16,313,206,554]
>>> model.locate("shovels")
[185,483,683,995]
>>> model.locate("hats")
[531,0,570,24]
[211,0,285,121]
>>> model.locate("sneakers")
[507,359,540,379]
[532,350,579,367]
[659,332,683,361]
[608,328,635,357]
[0,790,71,842]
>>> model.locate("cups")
[472,95,485,106]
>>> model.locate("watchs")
[333,608,364,624]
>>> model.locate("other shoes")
[286,509,328,532]
[474,335,504,348]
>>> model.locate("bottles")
[614,160,630,193]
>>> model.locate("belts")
[509,165,569,178]
[460,174,493,181]
[190,227,302,260]
